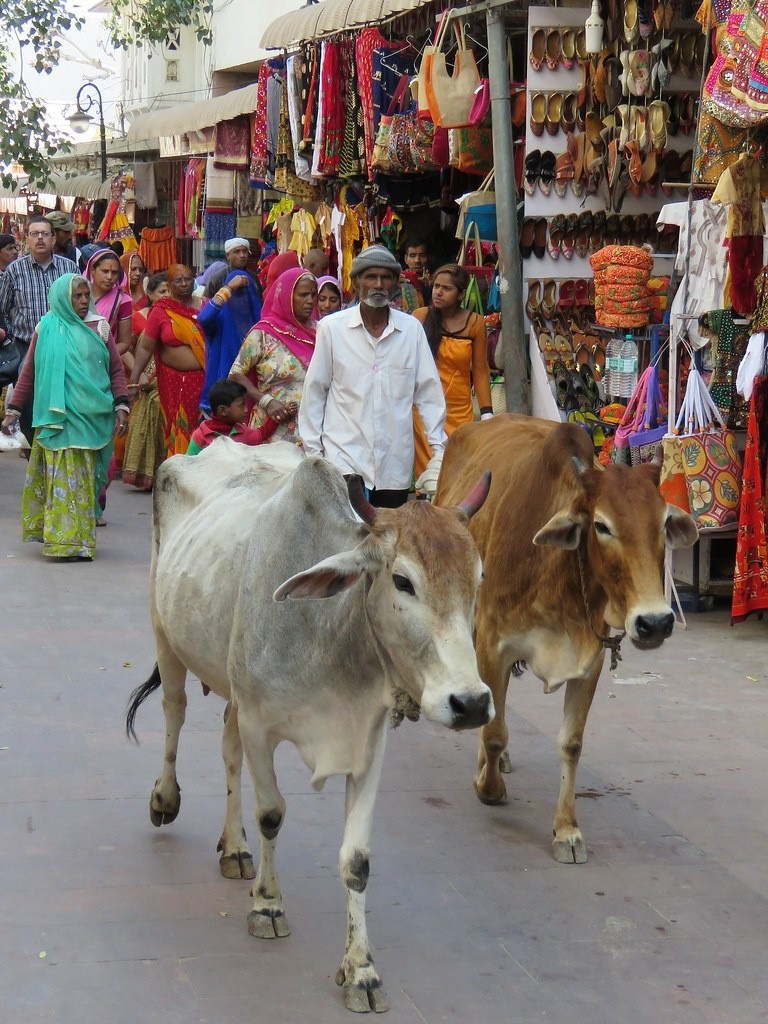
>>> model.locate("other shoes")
[521,1,705,410]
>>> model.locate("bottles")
[604,332,639,398]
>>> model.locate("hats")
[225,238,250,254]
[44,211,75,231]
[350,244,401,288]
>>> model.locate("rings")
[120,424,123,426]
[284,410,287,413]
[276,416,280,420]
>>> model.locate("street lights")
[65,83,106,182]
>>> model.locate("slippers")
[585,412,606,450]
[565,412,591,448]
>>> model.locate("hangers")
[445,23,488,66]
[414,28,433,74]
[147,218,166,229]
[380,35,423,75]
[299,20,389,52]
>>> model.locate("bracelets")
[114,405,130,415]
[216,288,231,302]
[259,394,274,409]
[127,384,139,388]
[5,411,16,417]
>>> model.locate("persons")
[296,244,451,506]
[0,213,438,560]
[407,264,495,501]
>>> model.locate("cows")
[434,409,678,865]
[125,433,496,1014]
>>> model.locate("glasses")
[27,231,52,238]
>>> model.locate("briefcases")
[611,362,744,534]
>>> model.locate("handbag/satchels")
[372,7,510,374]
[0,339,21,387]
